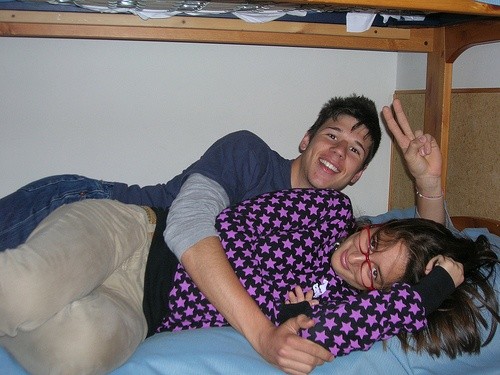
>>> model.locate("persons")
[0,187,500,375]
[0,95,446,375]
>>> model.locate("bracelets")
[415,191,442,200]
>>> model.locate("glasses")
[359,223,385,293]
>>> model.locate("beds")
[0,0,500,375]
